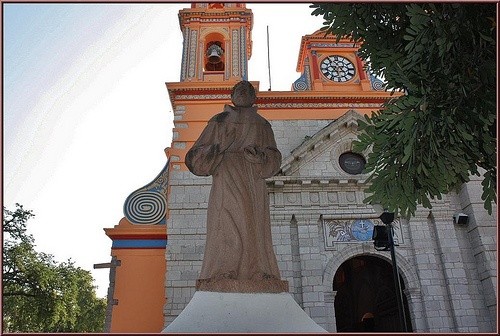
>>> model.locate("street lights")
[373,210,408,332]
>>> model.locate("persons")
[184,80,283,283]
